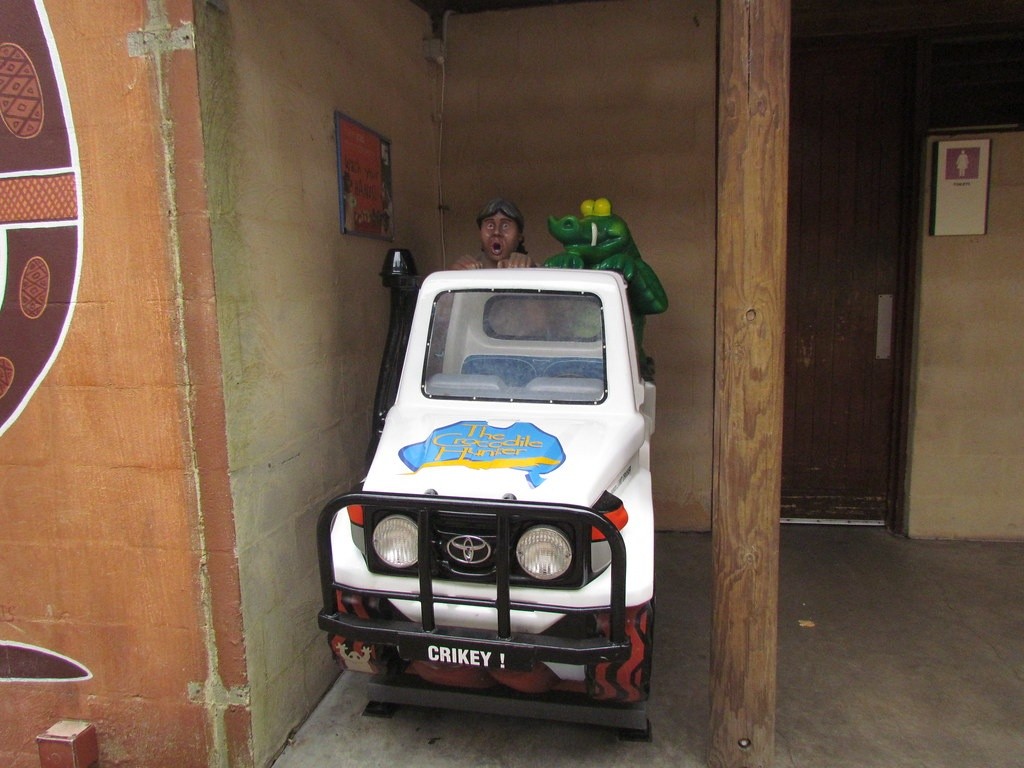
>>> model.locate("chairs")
[461,355,605,388]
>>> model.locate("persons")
[447,198,573,339]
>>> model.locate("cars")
[315,247,658,741]
